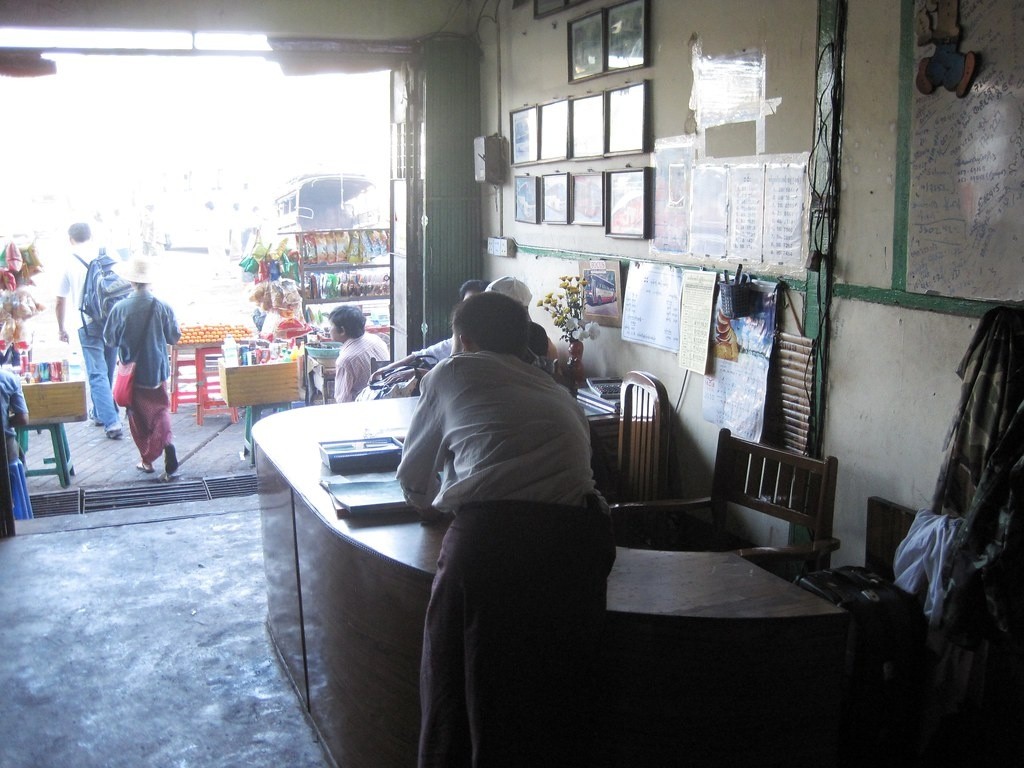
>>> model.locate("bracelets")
[414,368,422,379]
[58,330,67,335]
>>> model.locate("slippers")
[136,462,155,473]
[164,443,179,474]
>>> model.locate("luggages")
[793,565,927,768]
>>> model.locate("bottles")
[224,335,239,368]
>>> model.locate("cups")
[61,359,69,382]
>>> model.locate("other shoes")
[105,429,122,439]
[88,409,104,426]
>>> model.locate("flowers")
[535,275,601,343]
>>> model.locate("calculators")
[586,376,623,399]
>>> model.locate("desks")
[307,355,337,405]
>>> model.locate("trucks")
[265,173,383,250]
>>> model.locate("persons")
[329,305,391,403]
[396,292,616,768]
[55,222,123,438]
[364,276,563,386]
[0,349,29,473]
[102,259,181,474]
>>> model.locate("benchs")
[608,429,840,574]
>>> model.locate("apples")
[176,325,253,345]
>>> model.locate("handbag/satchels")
[113,357,137,409]
[355,354,439,402]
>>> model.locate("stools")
[243,402,291,465]
[15,422,75,488]
[170,346,239,425]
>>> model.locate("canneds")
[241,345,270,366]
[19,354,69,383]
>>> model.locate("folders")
[328,480,416,517]
[577,387,620,414]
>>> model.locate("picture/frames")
[541,172,569,226]
[604,167,651,240]
[509,103,538,168]
[603,79,649,158]
[568,90,605,162]
[513,175,539,224]
[533,0,593,20]
[536,96,569,163]
[567,7,604,85]
[603,0,651,75]
[570,171,603,226]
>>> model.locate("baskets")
[304,340,344,356]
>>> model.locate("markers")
[734,264,743,283]
[741,274,747,283]
[724,270,730,284]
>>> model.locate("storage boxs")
[21,363,88,425]
[217,358,299,408]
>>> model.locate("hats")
[111,254,161,284]
[484,275,533,307]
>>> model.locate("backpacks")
[890,306,1024,723]
[72,247,134,337]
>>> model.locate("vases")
[567,340,584,379]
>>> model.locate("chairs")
[370,357,391,376]
[614,370,670,552]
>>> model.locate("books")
[320,474,414,517]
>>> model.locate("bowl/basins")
[305,342,343,359]
